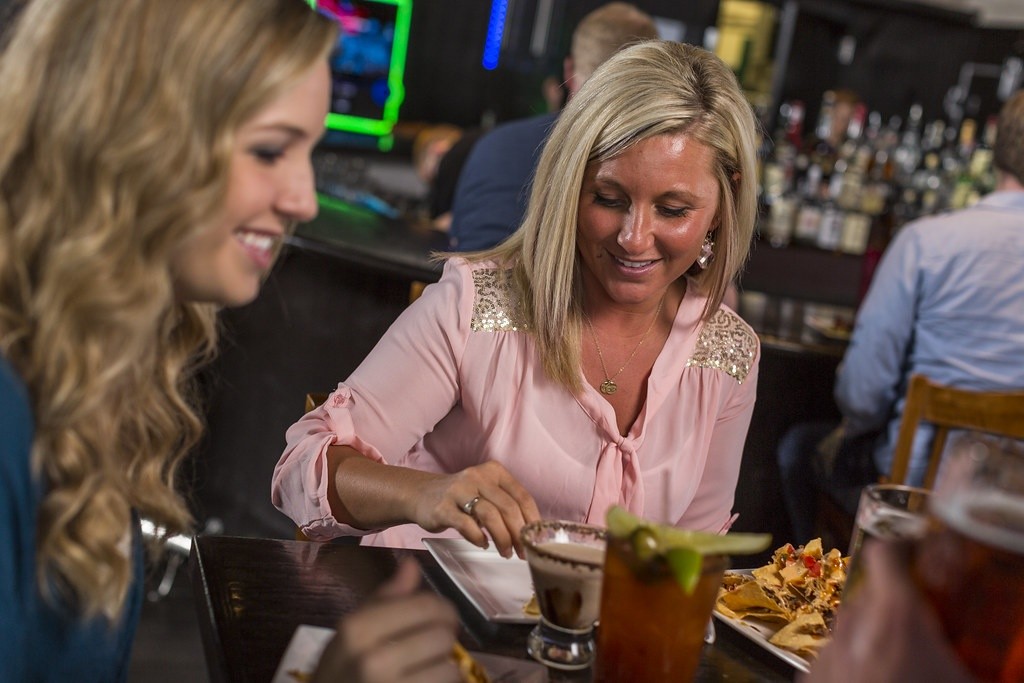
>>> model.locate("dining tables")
[188,532,797,682]
[283,215,858,362]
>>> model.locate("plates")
[712,568,811,674]
[422,537,542,623]
[272,624,549,683]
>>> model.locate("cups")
[595,533,725,683]
[913,428,1024,683]
[837,483,931,621]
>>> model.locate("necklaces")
[577,283,668,395]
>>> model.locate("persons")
[450,4,660,260]
[778,92,1024,545]
[4,1,462,683]
[272,43,761,556]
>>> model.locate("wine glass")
[521,519,608,670]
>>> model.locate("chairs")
[891,372,1023,515]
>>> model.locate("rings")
[462,494,484,514]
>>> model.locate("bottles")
[859,213,891,302]
[763,90,997,253]
